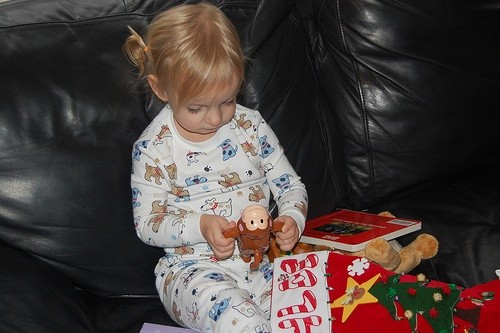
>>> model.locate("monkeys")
[222,205,285,271]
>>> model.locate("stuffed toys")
[290,211,438,274]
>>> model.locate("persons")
[122,3,309,333]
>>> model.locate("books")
[297,208,422,254]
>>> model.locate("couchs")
[0,0,500,333]
